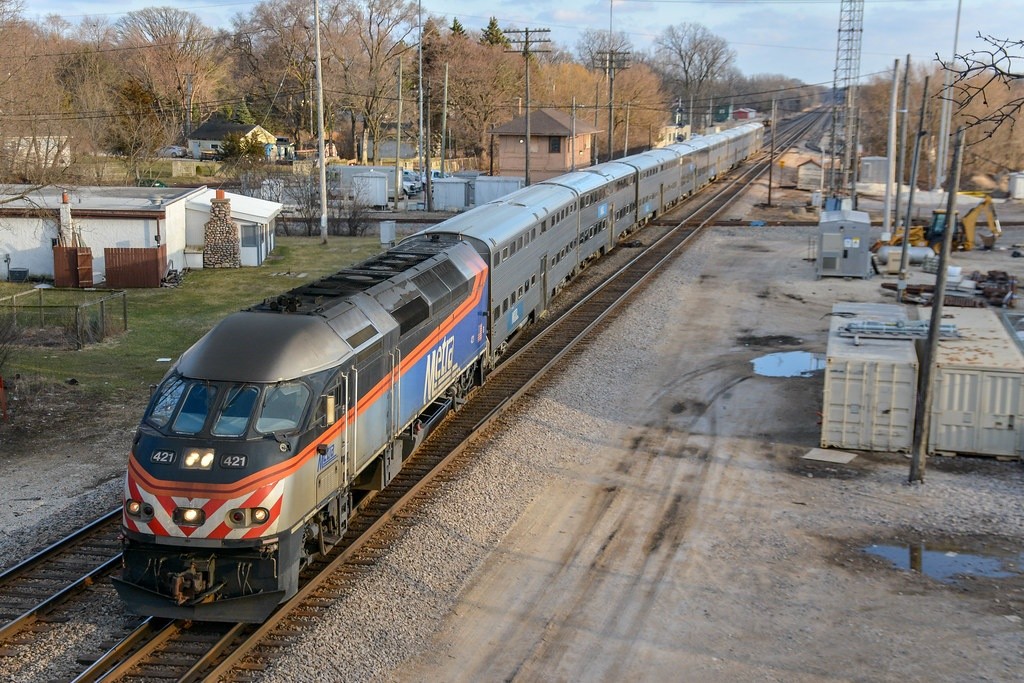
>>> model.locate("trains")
[106,121,765,625]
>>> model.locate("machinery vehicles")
[867,188,1004,254]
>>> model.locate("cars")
[403,168,450,198]
[135,178,167,188]
[153,145,188,158]
